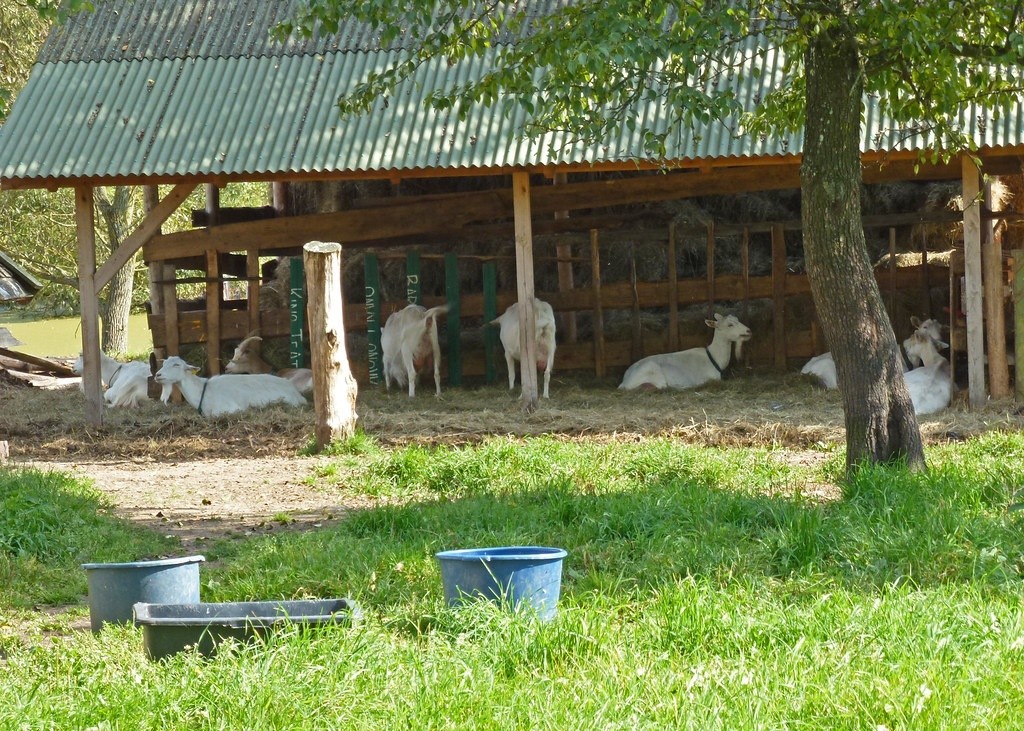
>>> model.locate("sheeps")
[379,303,451,400]
[70,347,154,409]
[154,336,314,424]
[800,315,960,417]
[489,296,557,401]
[616,313,752,393]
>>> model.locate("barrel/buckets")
[434,546,567,632]
[80,554,206,642]
